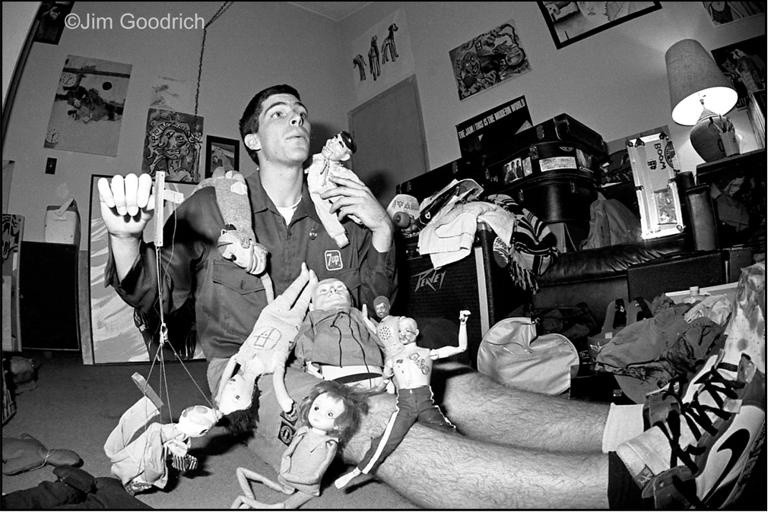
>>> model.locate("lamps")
[665,41,739,163]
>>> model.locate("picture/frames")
[206,135,238,181]
[535,1,663,50]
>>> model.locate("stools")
[534,237,680,313]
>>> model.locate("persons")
[191,164,268,275]
[97,83,767,508]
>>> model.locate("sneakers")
[615,262,766,508]
